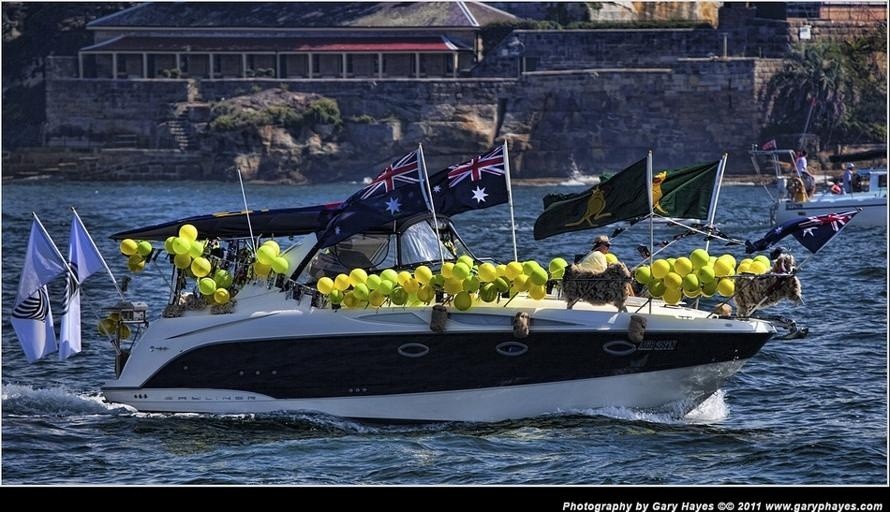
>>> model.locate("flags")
[743,206,862,255]
[652,152,728,230]
[8,210,69,365]
[58,206,105,365]
[531,148,656,242]
[422,137,513,223]
[316,141,436,251]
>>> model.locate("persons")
[843,163,855,194]
[790,149,800,171]
[795,150,815,197]
[832,179,841,194]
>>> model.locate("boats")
[102,168,886,425]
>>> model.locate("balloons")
[116,219,772,314]
